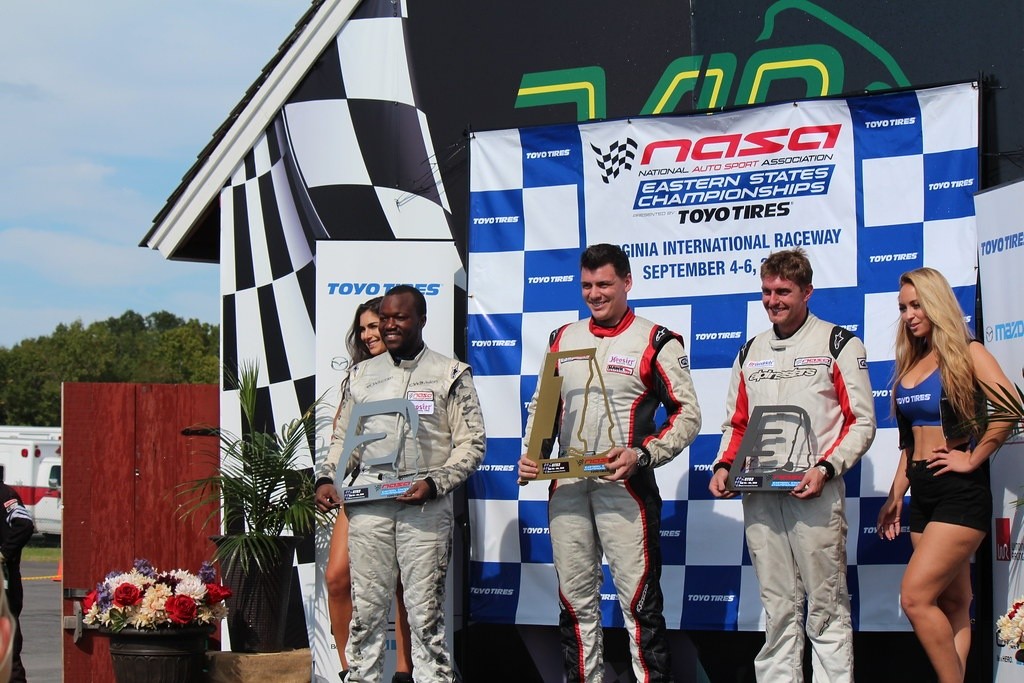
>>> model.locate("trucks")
[0,425,61,548]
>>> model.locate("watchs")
[817,465,829,478]
[633,447,648,469]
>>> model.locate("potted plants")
[162,359,337,655]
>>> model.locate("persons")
[325,296,412,682]
[516,243,703,683]
[707,249,878,683]
[313,284,488,682]
[0,482,35,683]
[877,268,1020,682]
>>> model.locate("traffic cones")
[53,556,62,581]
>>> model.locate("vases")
[109,628,209,683]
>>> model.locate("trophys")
[328,398,420,505]
[725,405,811,492]
[525,348,616,481]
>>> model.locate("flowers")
[995,597,1024,653]
[81,555,231,632]
